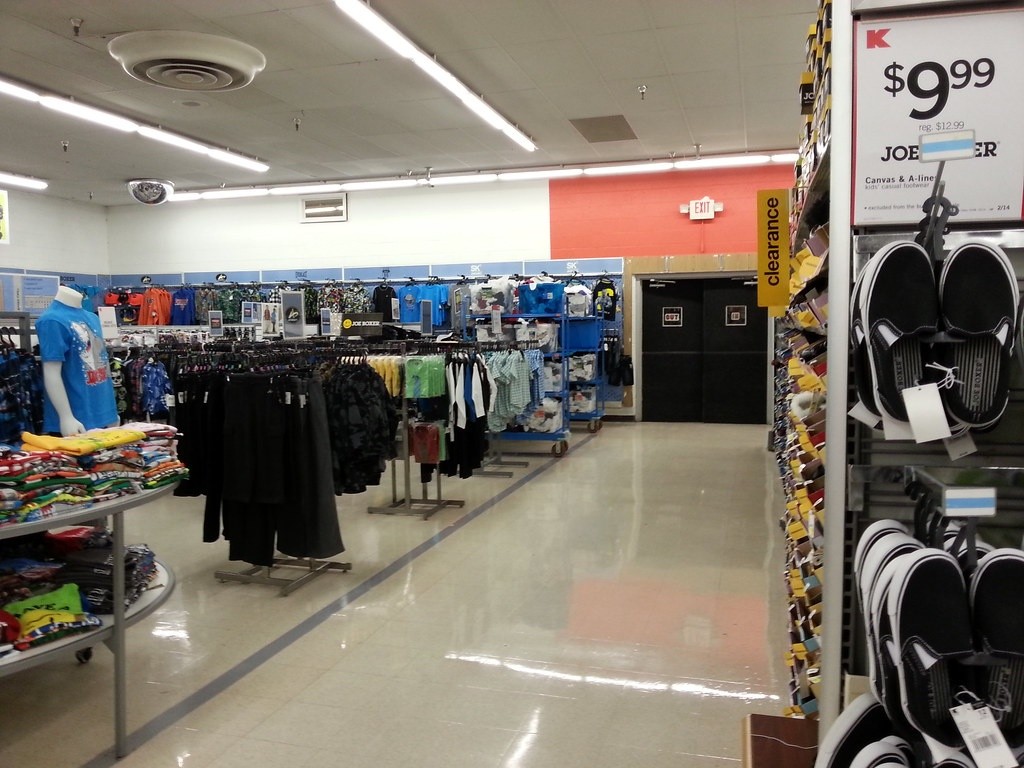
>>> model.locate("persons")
[263,305,270,333]
[271,305,278,334]
[34,287,120,437]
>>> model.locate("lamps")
[0,0,801,208]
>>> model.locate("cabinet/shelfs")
[0,481,181,758]
[462,298,570,458]
[566,301,605,433]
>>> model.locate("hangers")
[0,269,622,390]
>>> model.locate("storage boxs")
[769,0,833,721]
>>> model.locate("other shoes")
[812,236,1024,768]
